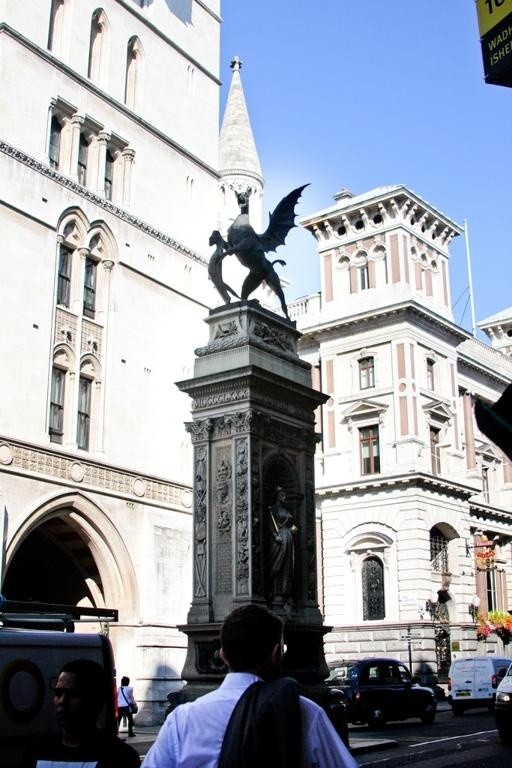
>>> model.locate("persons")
[139,602,360,767]
[18,657,141,768]
[116,673,138,737]
[267,487,297,603]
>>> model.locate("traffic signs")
[399,632,425,647]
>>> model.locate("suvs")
[324,656,437,727]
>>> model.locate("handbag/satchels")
[128,703,138,714]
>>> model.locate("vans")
[1,626,120,751]
[490,661,511,740]
[444,655,512,716]
